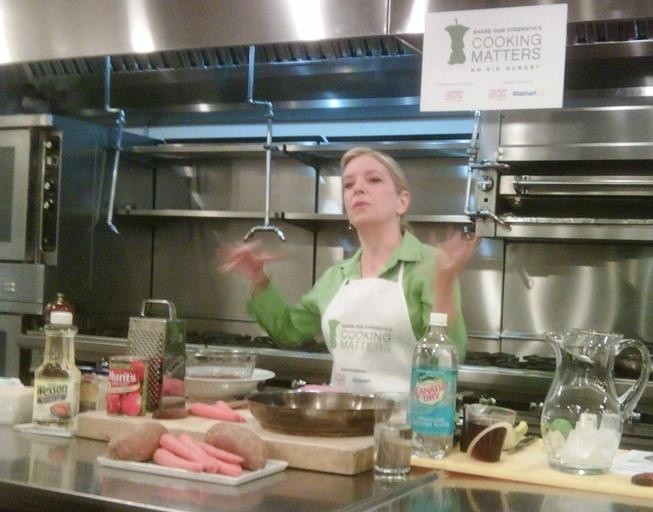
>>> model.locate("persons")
[218,147,480,423]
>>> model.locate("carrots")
[153,432,244,476]
[190,401,244,421]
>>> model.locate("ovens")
[0,113,163,385]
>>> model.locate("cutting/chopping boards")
[75,392,409,477]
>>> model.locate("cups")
[373,393,410,478]
[464,404,517,463]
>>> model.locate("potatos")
[205,422,267,471]
[107,421,167,462]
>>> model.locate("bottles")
[411,312,458,459]
[32,312,81,437]
[26,439,74,495]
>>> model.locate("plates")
[185,367,276,400]
[96,456,288,487]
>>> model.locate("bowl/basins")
[188,347,256,377]
[247,394,395,438]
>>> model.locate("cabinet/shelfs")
[114,144,477,225]
